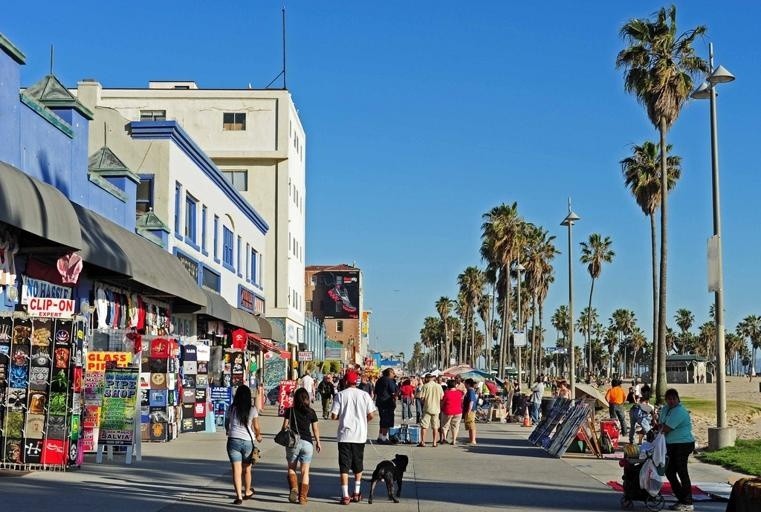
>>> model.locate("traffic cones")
[522,405,533,427]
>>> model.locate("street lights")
[400,352,406,375]
[557,195,582,403]
[421,283,479,372]
[509,252,528,393]
[688,39,734,426]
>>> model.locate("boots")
[299,483,309,504]
[288,474,298,502]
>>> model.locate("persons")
[293,366,571,447]
[224,384,263,504]
[331,369,378,505]
[281,387,321,506]
[586,368,696,511]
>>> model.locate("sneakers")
[353,492,361,502]
[670,503,694,511]
[340,497,350,504]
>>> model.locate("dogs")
[368,455,409,505]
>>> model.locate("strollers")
[616,421,667,511]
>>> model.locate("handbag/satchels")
[274,428,300,448]
[251,447,260,464]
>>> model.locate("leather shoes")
[245,488,254,499]
[234,500,241,504]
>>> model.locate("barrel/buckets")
[409,424,422,443]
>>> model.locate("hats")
[345,372,357,383]
